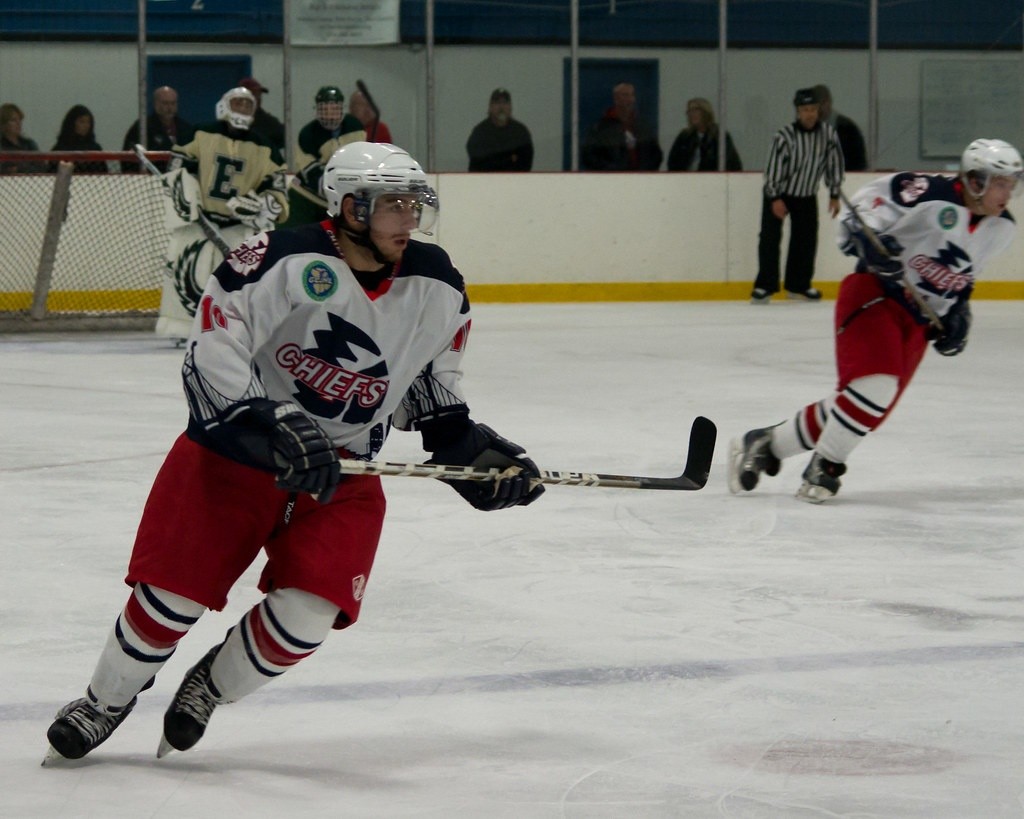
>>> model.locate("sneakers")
[728,419,787,494]
[158,624,236,759]
[749,288,771,303]
[40,671,156,766]
[795,451,846,504]
[786,287,820,302]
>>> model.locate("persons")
[466,88,533,173]
[667,96,742,171]
[815,84,869,172]
[120,86,197,173]
[750,88,846,305]
[0,103,46,172]
[47,104,109,173]
[348,90,392,143]
[591,83,664,171]
[285,86,367,226]
[39,141,546,767]
[725,140,1023,504]
[239,78,284,149]
[154,87,288,347]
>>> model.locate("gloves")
[861,234,904,282]
[420,421,545,511]
[934,307,972,357]
[218,394,338,500]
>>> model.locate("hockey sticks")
[823,139,946,332]
[355,79,382,142]
[132,144,232,257]
[339,412,721,495]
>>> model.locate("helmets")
[961,138,1023,196]
[326,142,429,218]
[216,87,257,130]
[315,86,344,130]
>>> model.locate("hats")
[792,88,819,106]
[489,88,510,101]
